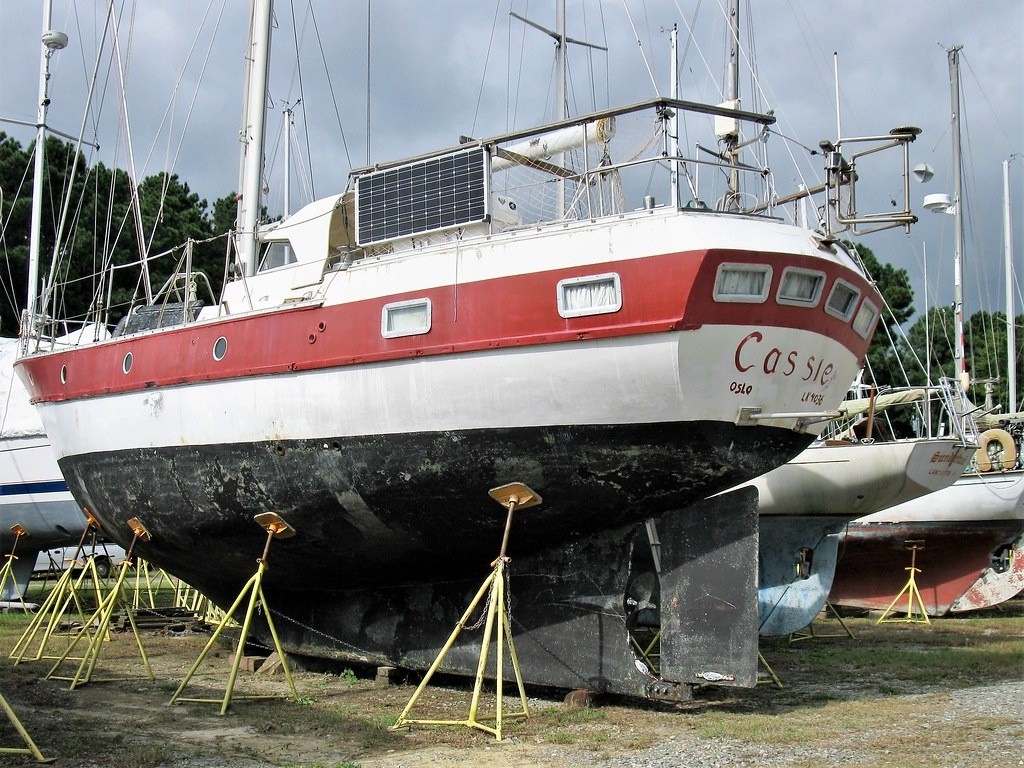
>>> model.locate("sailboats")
[0,1,1024,707]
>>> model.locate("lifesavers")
[975,427,1017,472]
